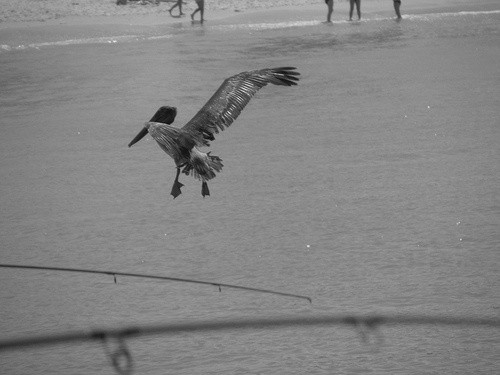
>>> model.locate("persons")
[394,0,402,19]
[169,0,186,16]
[191,0,205,23]
[324,0,335,22]
[349,0,361,21]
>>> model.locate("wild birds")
[129,66,302,198]
